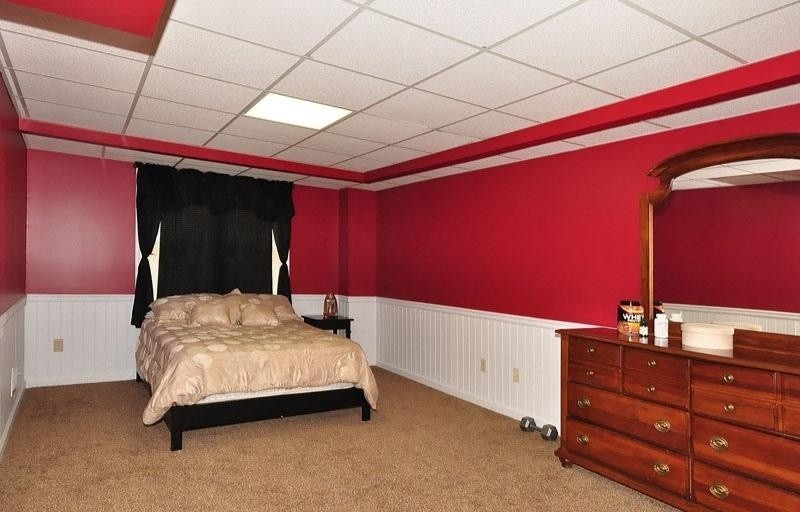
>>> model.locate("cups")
[323,312,329,320]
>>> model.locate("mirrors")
[639,131,800,355]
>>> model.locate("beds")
[134,290,375,452]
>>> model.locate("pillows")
[149,292,303,329]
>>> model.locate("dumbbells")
[520,416,557,440]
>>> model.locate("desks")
[304,316,354,340]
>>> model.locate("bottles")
[653,313,669,339]
[323,289,339,317]
[618,300,645,335]
[638,318,648,336]
[653,301,666,319]
[654,336,668,348]
[640,334,649,345]
[670,313,683,323]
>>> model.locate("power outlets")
[53,339,64,352]
[11,366,19,396]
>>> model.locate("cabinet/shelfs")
[553,321,800,512]
